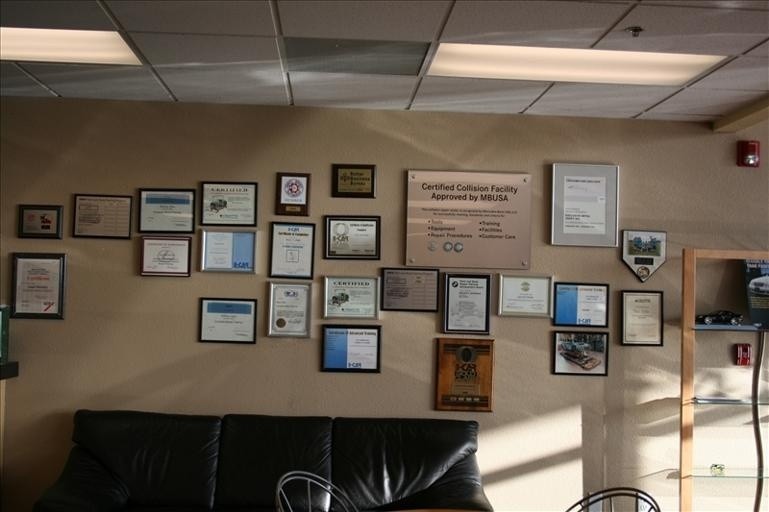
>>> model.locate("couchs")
[37,408,496,511]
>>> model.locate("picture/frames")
[621,289,664,347]
[550,163,619,249]
[621,229,667,284]
[444,272,492,336]
[201,180,258,227]
[138,187,196,235]
[551,331,609,376]
[496,272,554,320]
[140,235,191,278]
[275,170,310,217]
[269,221,317,280]
[16,202,64,241]
[552,281,610,330]
[201,229,257,274]
[331,164,377,199]
[266,280,312,340]
[379,266,438,310]
[10,251,66,321]
[72,192,132,240]
[198,296,258,345]
[319,323,382,374]
[324,215,382,262]
[432,336,494,413]
[323,274,380,320]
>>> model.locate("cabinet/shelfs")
[689,313,769,479]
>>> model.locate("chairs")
[274,471,364,512]
[564,487,661,512]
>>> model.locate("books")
[742,260,769,330]
